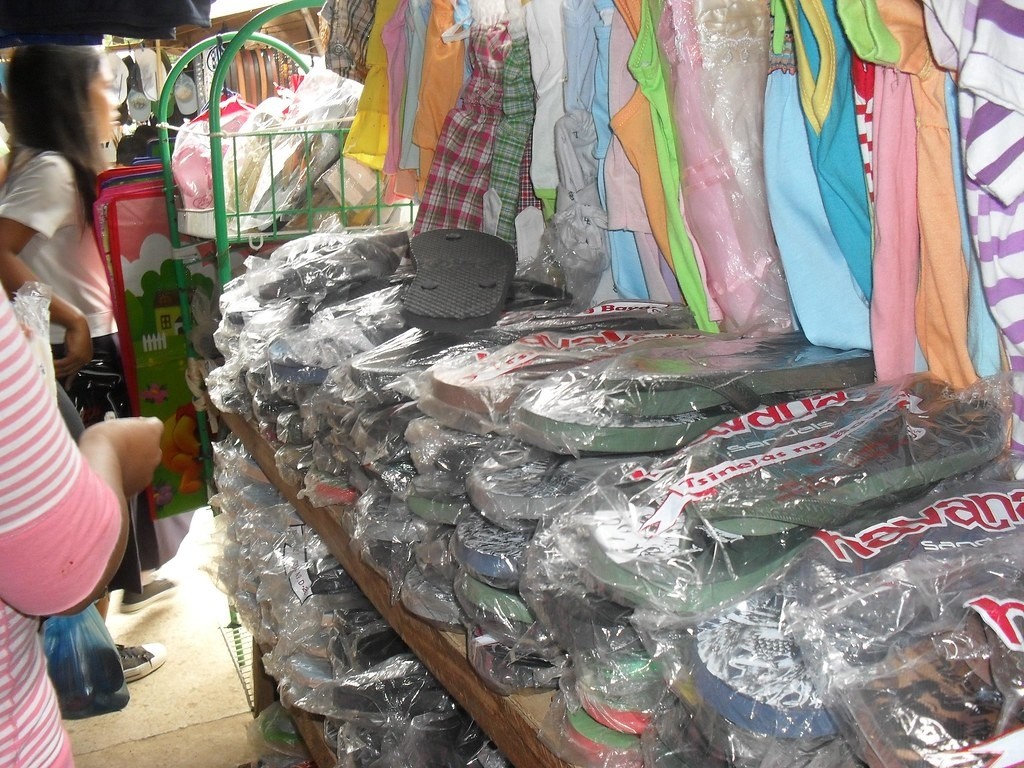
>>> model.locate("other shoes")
[120,579,179,612]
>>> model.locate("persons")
[0,42,165,768]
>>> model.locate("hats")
[99,49,198,121]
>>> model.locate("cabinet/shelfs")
[203,390,572,768]
[155,1,417,669]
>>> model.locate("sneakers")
[115,643,167,683]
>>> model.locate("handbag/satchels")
[59,360,128,429]
[38,602,130,720]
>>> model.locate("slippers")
[206,227,1023,768]
